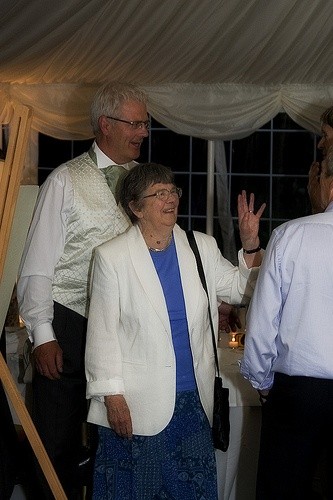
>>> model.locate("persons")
[85,162,267,500]
[238,99,333,500]
[14,80,149,500]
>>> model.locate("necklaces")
[141,231,172,253]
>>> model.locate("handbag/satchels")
[213,377,229,452]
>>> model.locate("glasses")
[107,115,151,129]
[143,187,182,200]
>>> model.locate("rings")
[248,210,253,213]
[241,246,263,256]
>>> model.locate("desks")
[215,326,264,500]
[6,326,27,425]
